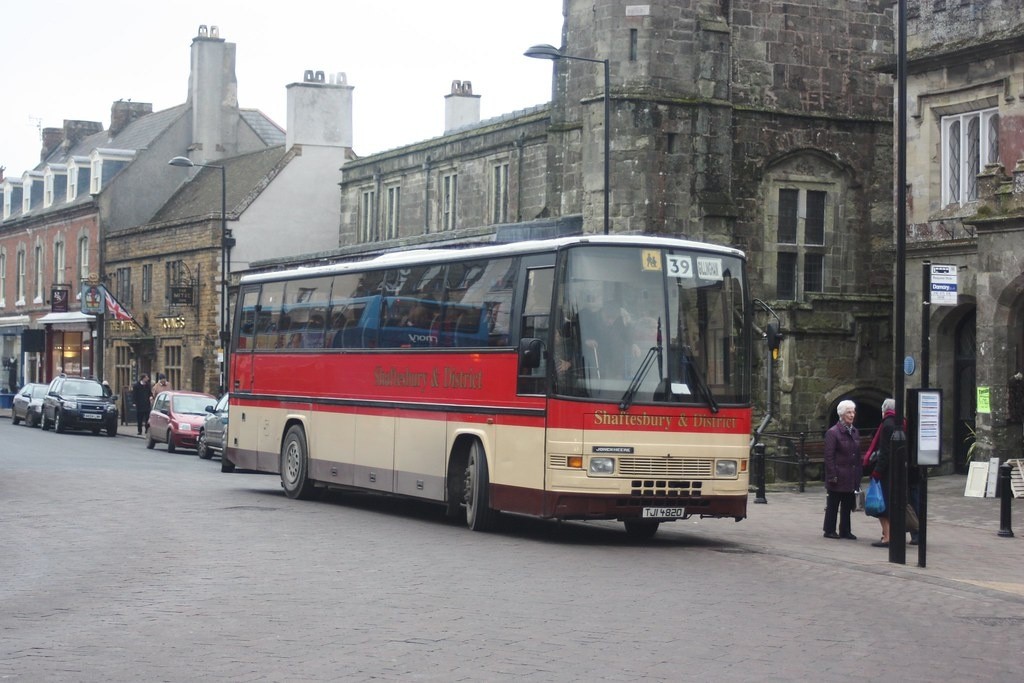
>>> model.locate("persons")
[248,295,659,396]
[823,400,860,539]
[151,374,172,404]
[133,373,152,434]
[864,398,919,546]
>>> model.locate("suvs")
[40,372,120,437]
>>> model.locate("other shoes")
[839,529,856,540]
[871,537,891,547]
[824,531,840,539]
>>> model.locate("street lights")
[522,45,612,235]
[166,153,230,395]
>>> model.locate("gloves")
[828,477,838,485]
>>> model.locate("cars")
[197,391,230,459]
[145,389,220,452]
[11,382,50,428]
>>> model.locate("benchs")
[797,434,875,482]
[625,343,682,381]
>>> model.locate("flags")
[105,290,132,319]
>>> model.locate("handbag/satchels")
[904,504,919,533]
[864,477,886,516]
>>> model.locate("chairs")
[427,310,469,348]
[533,309,565,354]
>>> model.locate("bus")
[225,233,783,538]
